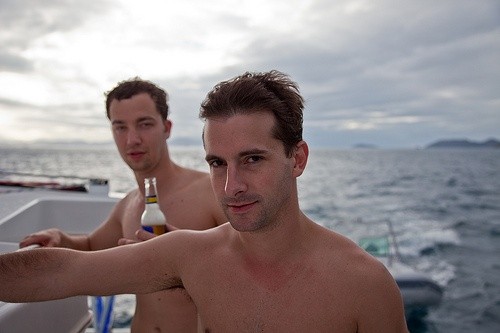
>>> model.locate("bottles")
[139,175,167,239]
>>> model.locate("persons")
[18,81,230,332]
[0,71,409,333]
